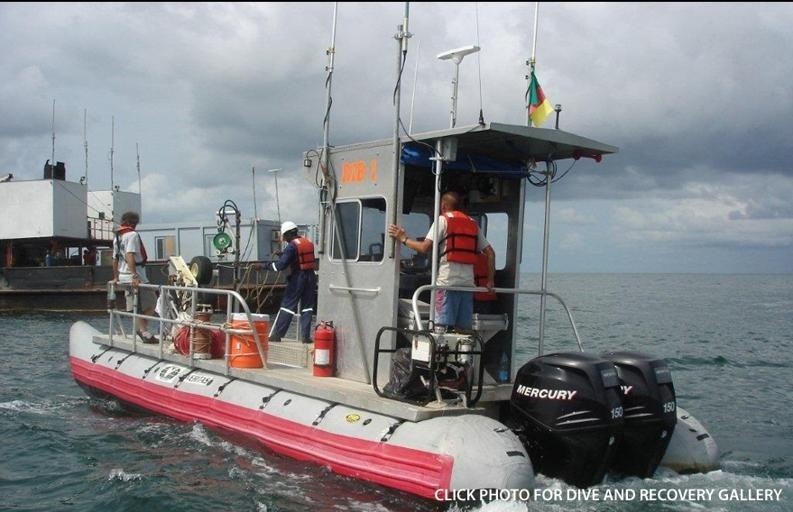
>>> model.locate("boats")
[0,101,319,308]
[68,1,719,502]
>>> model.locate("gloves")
[253,264,262,270]
[275,249,281,255]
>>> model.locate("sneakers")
[137,328,159,344]
[302,338,312,343]
[268,337,281,342]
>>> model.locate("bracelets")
[402,237,408,246]
[131,273,139,279]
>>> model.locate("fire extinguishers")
[312,320,337,378]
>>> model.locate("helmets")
[281,221,298,235]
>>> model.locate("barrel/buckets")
[229,314,271,369]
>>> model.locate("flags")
[529,71,552,129]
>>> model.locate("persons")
[255,220,316,343]
[113,213,161,345]
[472,255,497,315]
[388,192,496,336]
[82,245,97,266]
